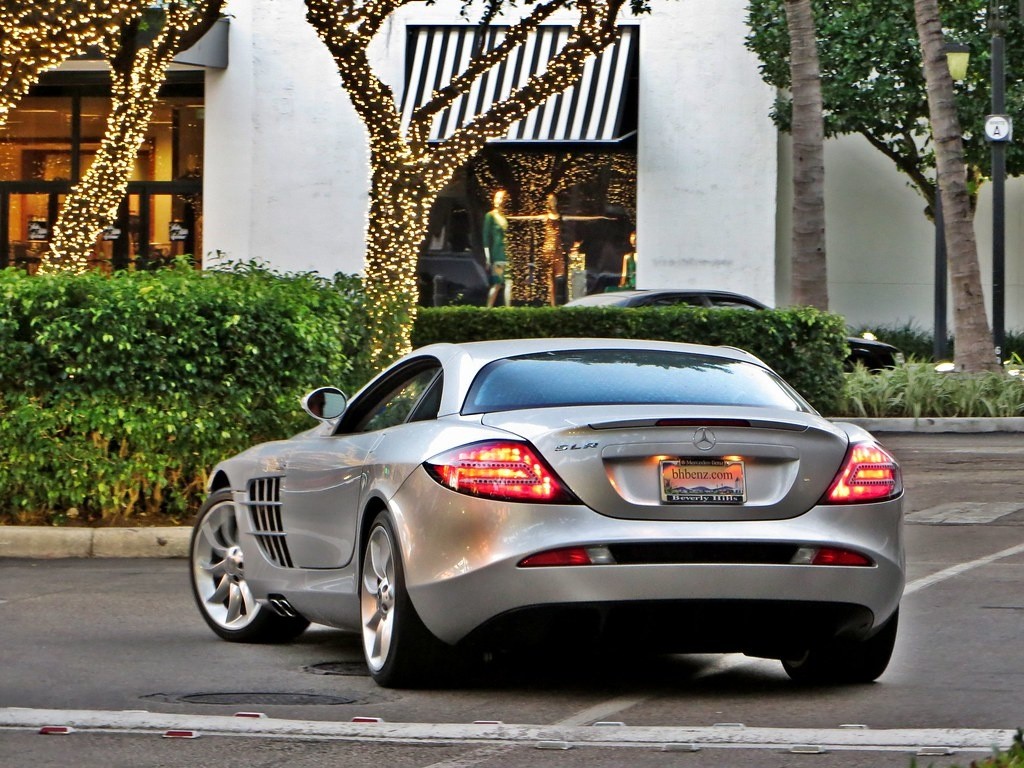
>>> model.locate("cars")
[560,289,904,375]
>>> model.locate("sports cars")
[188,337,907,692]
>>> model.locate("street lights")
[931,42,970,360]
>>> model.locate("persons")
[604,231,636,292]
[482,190,513,307]
[506,194,617,307]
